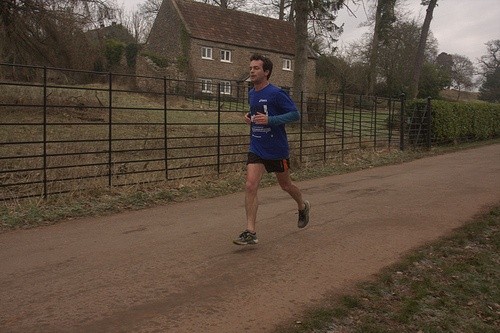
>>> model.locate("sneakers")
[233,231,258,244]
[298,200,311,230]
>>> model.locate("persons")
[232,52,310,245]
